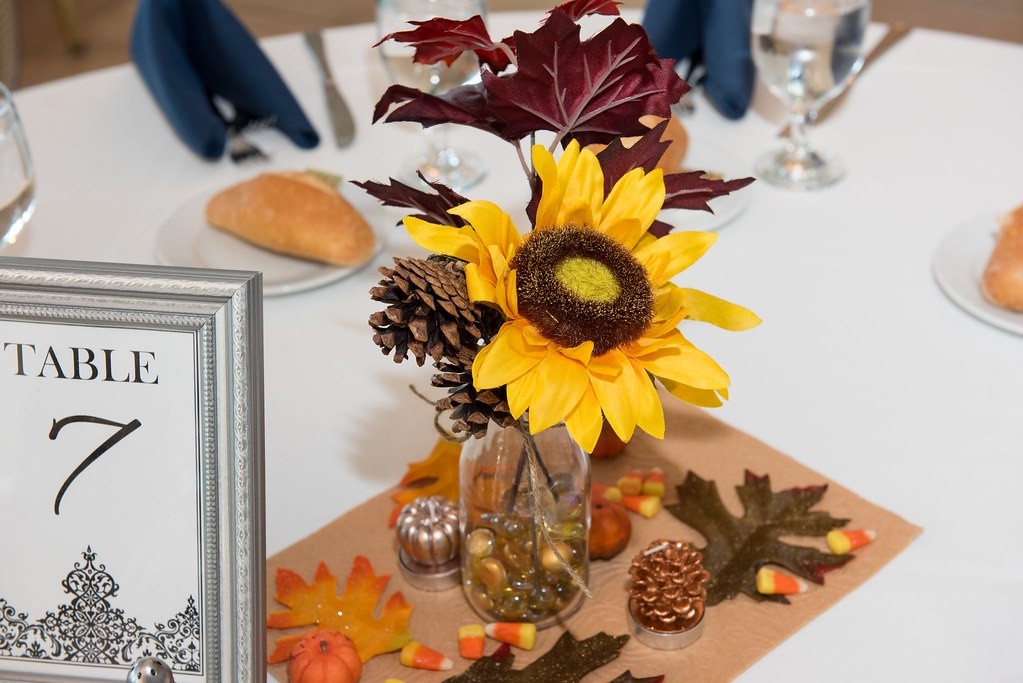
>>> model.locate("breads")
[208,170,378,266]
[984,203,1023,312]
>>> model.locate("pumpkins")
[287,629,363,683]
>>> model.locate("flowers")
[347,1,758,455]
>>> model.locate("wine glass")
[374,0,491,192]
[748,0,871,189]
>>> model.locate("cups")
[0,80,37,253]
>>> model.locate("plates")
[932,211,1022,337]
[658,182,749,232]
[157,169,384,299]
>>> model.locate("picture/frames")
[0,257,272,683]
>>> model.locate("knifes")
[302,24,358,148]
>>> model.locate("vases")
[455,411,589,624]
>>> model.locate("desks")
[0,14,1023,683]
